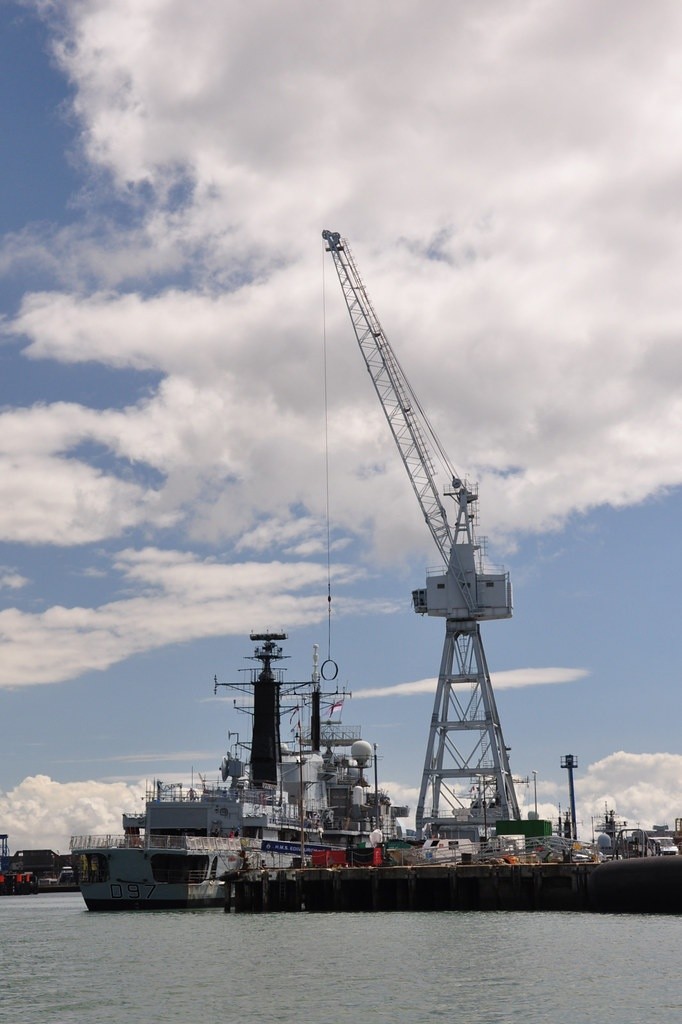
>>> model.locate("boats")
[2,629,408,912]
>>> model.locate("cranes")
[320,226,531,846]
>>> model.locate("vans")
[647,836,679,857]
